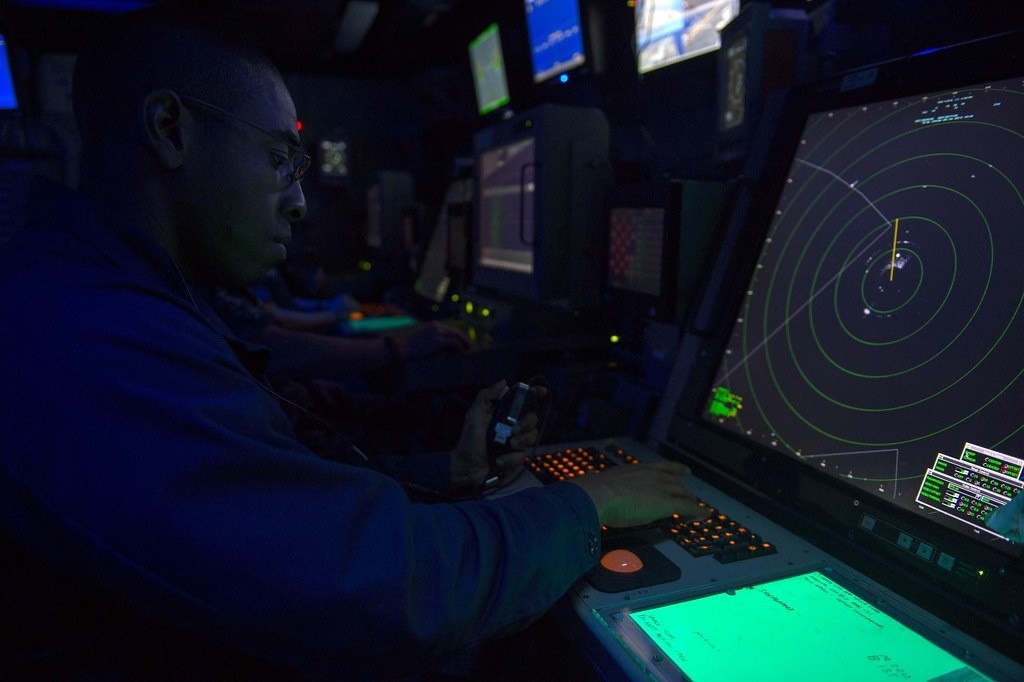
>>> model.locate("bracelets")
[384,336,399,362]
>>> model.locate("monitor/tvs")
[467,0,740,115]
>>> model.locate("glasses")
[169,93,311,187]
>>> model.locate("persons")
[0,6,711,682]
[195,267,473,379]
